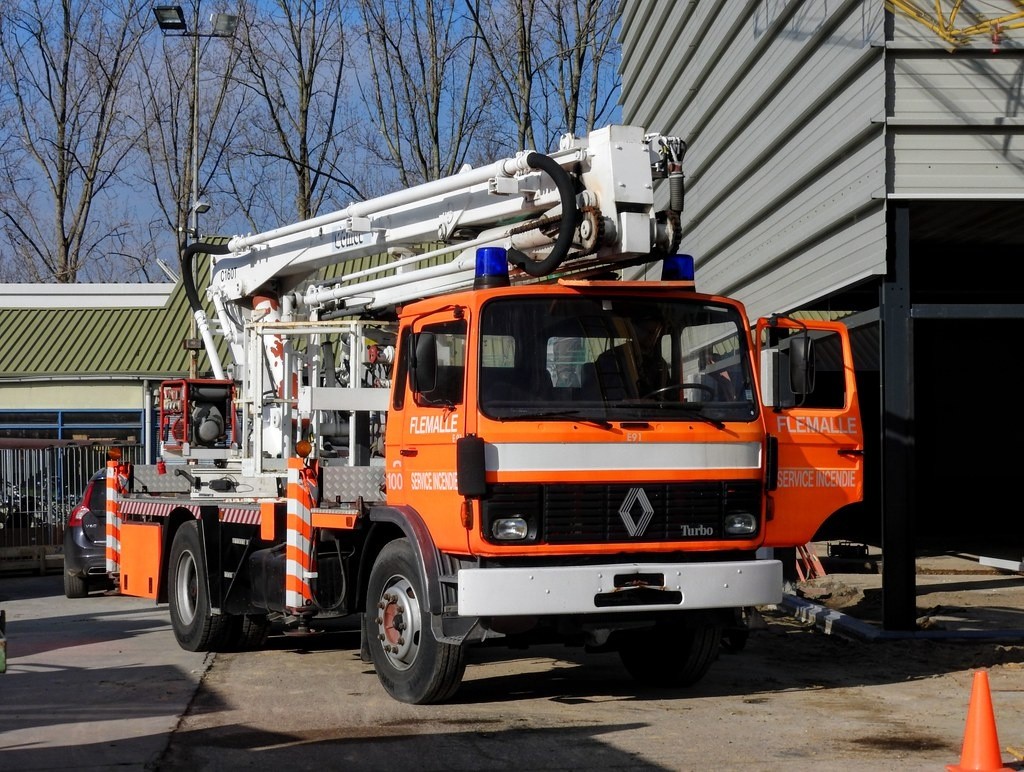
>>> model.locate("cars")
[0,459,100,527]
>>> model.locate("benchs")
[427,365,553,403]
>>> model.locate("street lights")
[151,4,239,376]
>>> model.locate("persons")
[595,303,678,401]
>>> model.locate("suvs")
[64,467,108,598]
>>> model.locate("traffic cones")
[945,671,1018,772]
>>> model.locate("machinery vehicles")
[105,125,865,706]
